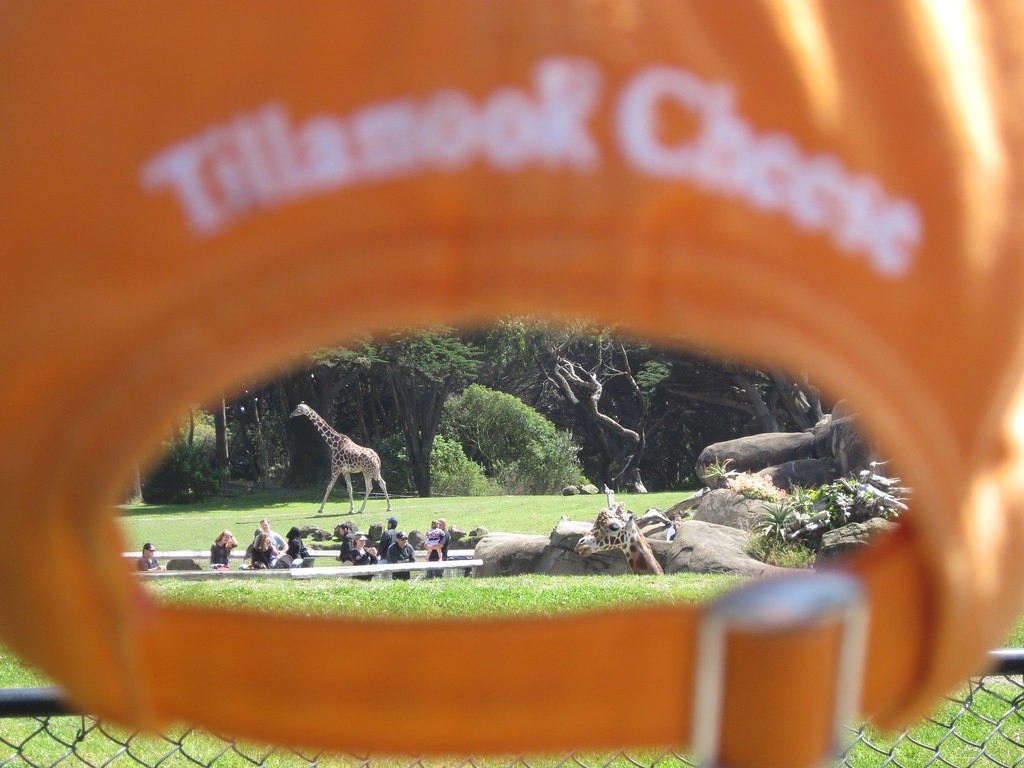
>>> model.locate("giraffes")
[574,504,663,575]
[289,401,392,514]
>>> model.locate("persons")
[209,530,238,570]
[246,518,311,569]
[137,543,167,571]
[336,517,416,580]
[421,518,451,578]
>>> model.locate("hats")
[254,528,262,536]
[395,532,406,538]
[387,517,397,525]
[353,531,367,540]
[0,0,1024,768]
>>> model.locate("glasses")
[399,537,407,541]
[146,549,155,552]
